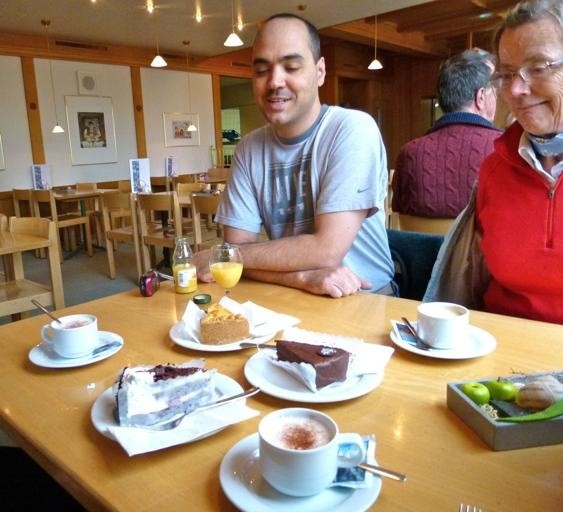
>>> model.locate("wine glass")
[209,244,243,298]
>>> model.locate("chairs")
[386,213,456,236]
[386,228,444,301]
[1,166,230,320]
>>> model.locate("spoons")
[401,315,433,352]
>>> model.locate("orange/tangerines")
[460,381,490,406]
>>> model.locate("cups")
[259,408,366,498]
[416,302,470,349]
[41,315,98,358]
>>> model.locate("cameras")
[140,269,160,297]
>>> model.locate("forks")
[135,387,260,432]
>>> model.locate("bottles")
[173,236,197,294]
[194,294,211,313]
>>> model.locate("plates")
[169,322,278,352]
[220,432,383,511]
[28,331,124,368]
[391,321,496,359]
[90,373,245,443]
[244,346,386,402]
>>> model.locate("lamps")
[223,0,244,47]
[150,38,168,68]
[367,16,383,70]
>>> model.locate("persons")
[390,45,507,218]
[188,12,399,298]
[475,0,563,326]
[83,121,102,148]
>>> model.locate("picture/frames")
[162,111,200,147]
[63,95,118,166]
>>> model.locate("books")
[128,157,152,193]
[166,152,176,177]
[31,164,52,191]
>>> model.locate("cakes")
[200,301,250,345]
[275,338,351,394]
[113,361,218,429]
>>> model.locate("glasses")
[489,59,563,89]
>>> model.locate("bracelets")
[220,241,230,262]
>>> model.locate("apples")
[487,376,516,401]
